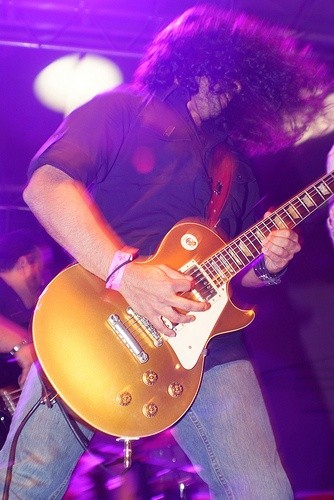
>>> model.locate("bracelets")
[8,337,34,358]
[255,256,291,287]
[104,250,132,291]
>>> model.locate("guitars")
[28,168,334,442]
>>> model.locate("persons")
[0,0,302,500]
